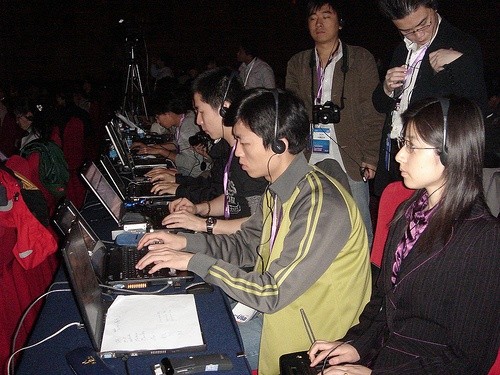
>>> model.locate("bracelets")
[344,373,348,375]
[202,200,210,215]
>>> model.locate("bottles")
[109,142,118,163]
[126,131,133,149]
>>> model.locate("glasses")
[400,8,431,42]
[397,137,440,153]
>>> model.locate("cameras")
[311,101,340,124]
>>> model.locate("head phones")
[219,75,234,118]
[437,96,449,167]
[271,89,286,155]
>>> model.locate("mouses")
[186,281,213,295]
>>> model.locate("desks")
[15,192,253,374]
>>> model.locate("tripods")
[122,46,149,122]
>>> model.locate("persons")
[147,139,233,204]
[129,88,212,177]
[372,0,492,214]
[308,97,500,375]
[0,78,101,214]
[235,38,275,89]
[135,88,372,374]
[284,0,385,248]
[162,65,268,235]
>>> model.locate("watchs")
[206,216,217,234]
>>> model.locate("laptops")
[52,111,207,359]
